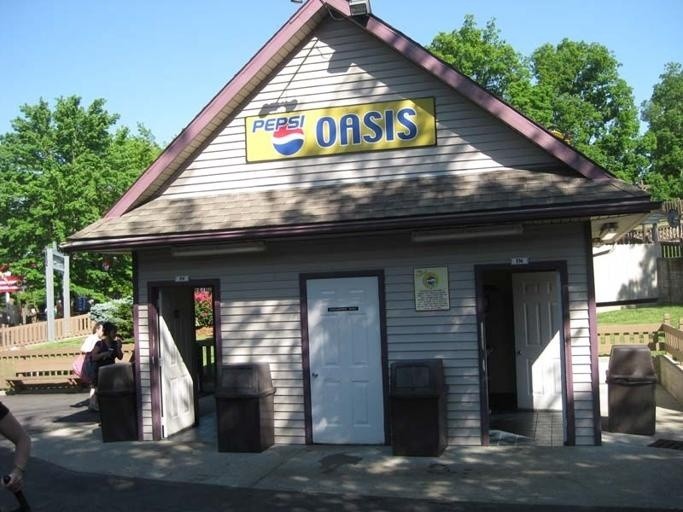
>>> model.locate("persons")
[5,297,63,328]
[78,321,104,402]
[0,401,31,496]
[87,321,123,413]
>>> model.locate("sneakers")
[87,405,100,413]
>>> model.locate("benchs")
[5,359,90,394]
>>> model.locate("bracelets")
[14,463,26,473]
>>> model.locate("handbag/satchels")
[72,357,85,376]
[79,351,97,385]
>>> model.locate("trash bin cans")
[97,362,138,443]
[215,361,276,453]
[387,360,449,457]
[605,345,660,436]
